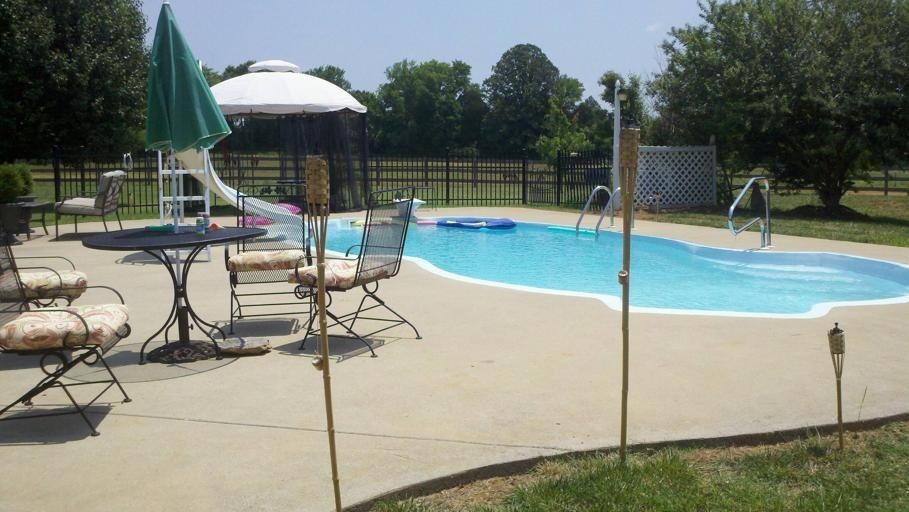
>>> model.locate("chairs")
[225,184,314,335]
[0,224,88,314]
[288,186,423,358]
[0,286,132,436]
[54,170,127,241]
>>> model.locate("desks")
[82,226,267,365]
[277,180,306,205]
[3,201,54,240]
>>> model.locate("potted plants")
[1,163,34,245]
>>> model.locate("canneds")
[196,211,211,236]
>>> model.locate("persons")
[142,2,233,307]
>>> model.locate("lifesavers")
[238,202,301,225]
[437,218,515,229]
